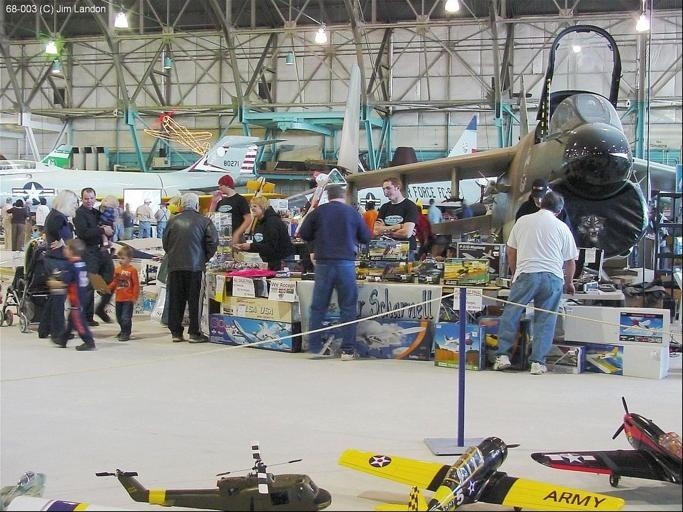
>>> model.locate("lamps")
[286,51,295,64]
[163,44,172,69]
[51,57,60,75]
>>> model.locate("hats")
[218,175,234,187]
[531,179,547,197]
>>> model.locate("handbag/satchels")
[157,263,168,284]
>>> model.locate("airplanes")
[623,318,657,334]
[226,318,293,351]
[442,333,474,346]
[586,346,622,374]
[318,326,424,358]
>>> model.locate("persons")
[515,178,572,233]
[1,174,472,360]
[493,192,579,375]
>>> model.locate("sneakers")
[530,362,547,374]
[95,307,110,322]
[312,346,334,358]
[116,332,129,341]
[188,334,208,343]
[160,324,167,328]
[99,241,113,253]
[493,355,512,370]
[341,351,360,361]
[39,320,99,350]
[172,334,183,342]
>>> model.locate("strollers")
[0,233,55,332]
